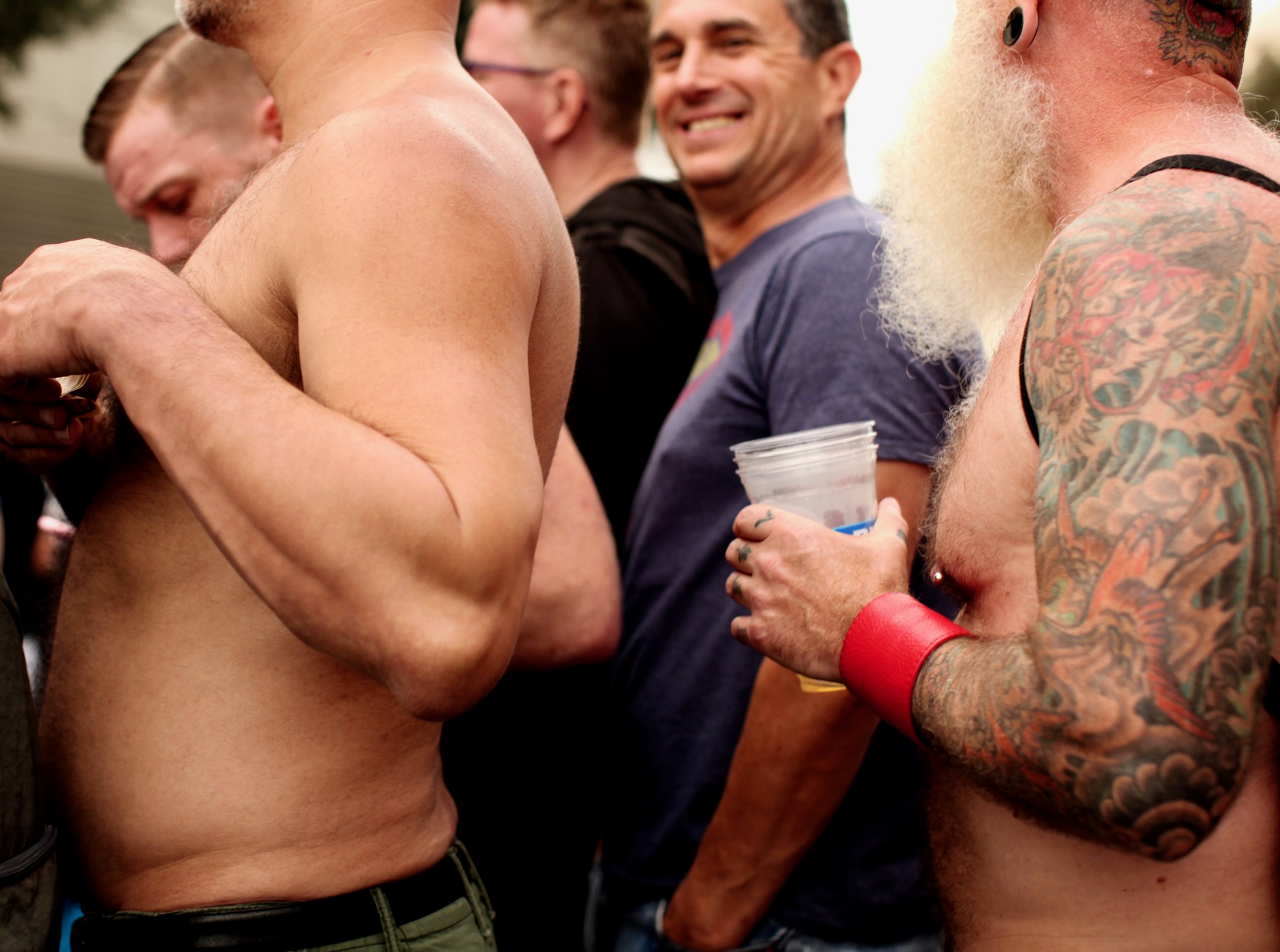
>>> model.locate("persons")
[414,0,759,952]
[0,0,626,952]
[581,0,993,952]
[80,11,300,277]
[728,0,1280,952]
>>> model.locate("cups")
[727,420,878,691]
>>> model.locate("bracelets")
[838,591,971,753]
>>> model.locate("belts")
[68,843,470,952]
